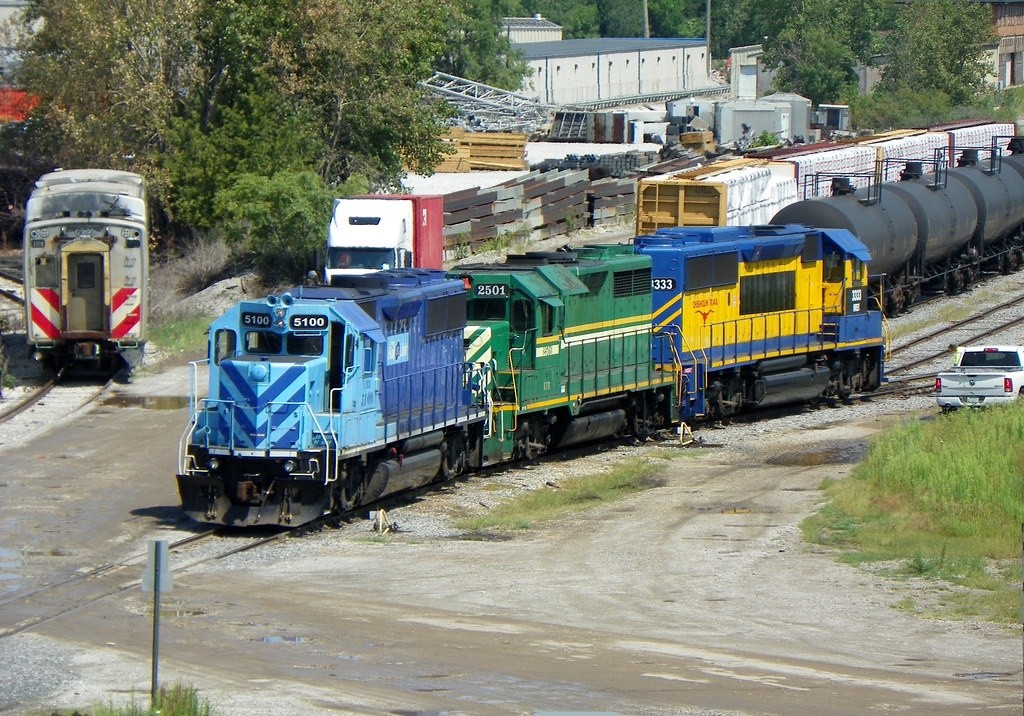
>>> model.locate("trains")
[176,224,889,530]
[634,120,1014,232]
[24,167,149,380]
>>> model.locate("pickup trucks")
[935,344,1024,414]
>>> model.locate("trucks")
[308,194,444,287]
